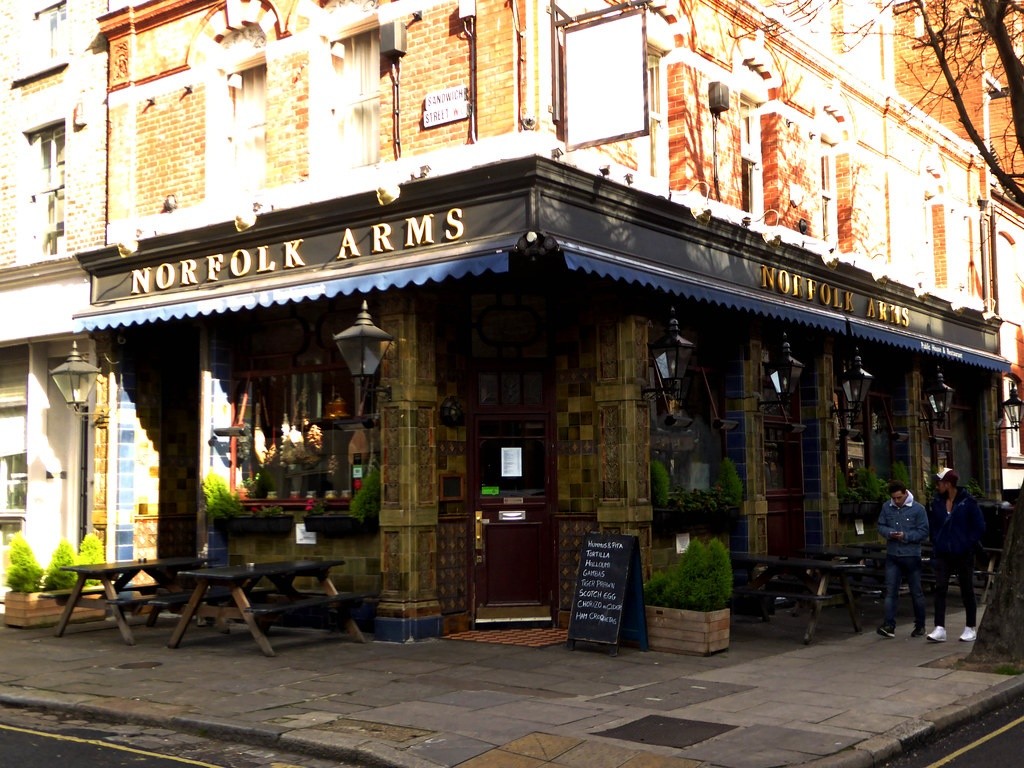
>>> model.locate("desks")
[742,542,988,645]
[163,557,365,657]
[53,556,217,646]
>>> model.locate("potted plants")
[5,532,109,626]
[642,541,738,654]
[835,463,940,518]
[647,454,742,536]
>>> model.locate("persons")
[876,479,929,638]
[926,467,987,642]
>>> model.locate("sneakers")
[878,623,895,638]
[927,626,946,642]
[911,624,927,637]
[959,627,976,641]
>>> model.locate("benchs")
[40,585,372,616]
[729,562,1005,606]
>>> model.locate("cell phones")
[890,531,898,535]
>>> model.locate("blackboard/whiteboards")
[567,533,647,645]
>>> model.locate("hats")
[931,467,958,482]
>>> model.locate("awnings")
[548,235,1012,373]
[73,232,522,332]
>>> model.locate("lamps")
[48,341,124,432]
[908,270,932,302]
[828,347,878,425]
[945,290,968,315]
[916,363,957,427]
[978,300,1004,325]
[671,179,713,225]
[642,306,698,429]
[117,227,158,256]
[233,199,277,232]
[332,296,397,398]
[624,173,634,186]
[375,166,432,206]
[551,147,563,161]
[994,383,1024,430]
[756,331,807,409]
[864,252,893,286]
[802,231,843,270]
[743,209,781,249]
[598,163,610,178]
